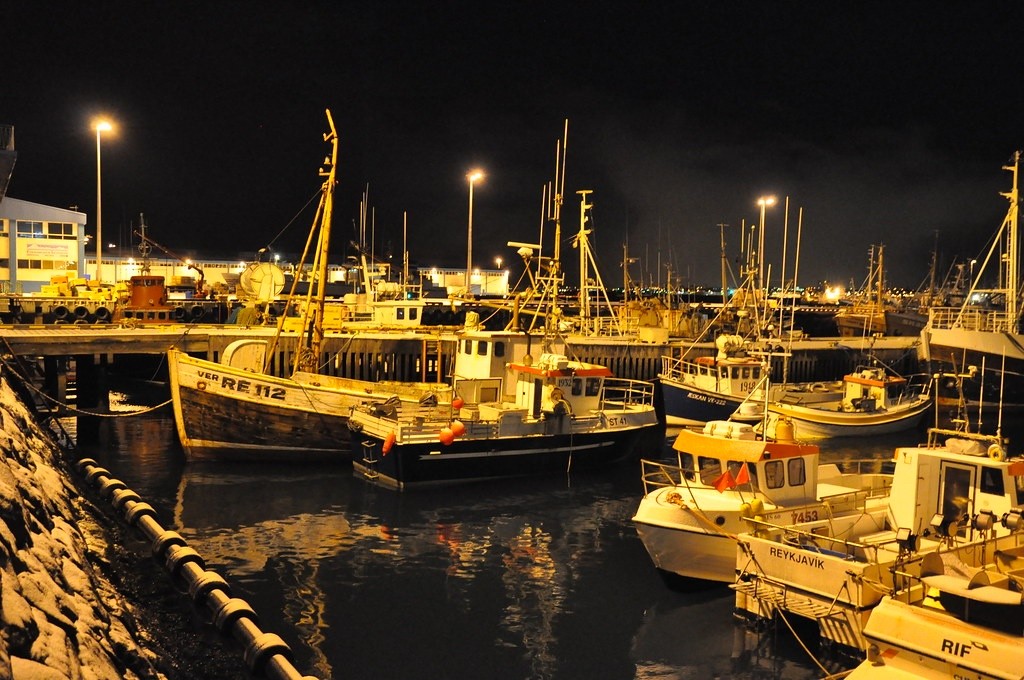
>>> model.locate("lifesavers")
[73,306,89,319]
[95,320,110,324]
[54,319,69,324]
[192,305,205,318]
[810,382,826,392]
[73,319,88,324]
[53,306,68,319]
[174,306,186,319]
[95,306,110,320]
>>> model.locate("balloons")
[452,419,465,436]
[440,428,455,445]
[452,396,464,409]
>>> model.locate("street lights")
[968,259,977,306]
[757,196,774,302]
[463,171,482,292]
[94,122,112,283]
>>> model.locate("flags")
[735,463,750,486]
[711,472,736,493]
[1007,460,1024,476]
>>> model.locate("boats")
[0,106,1023,680]
